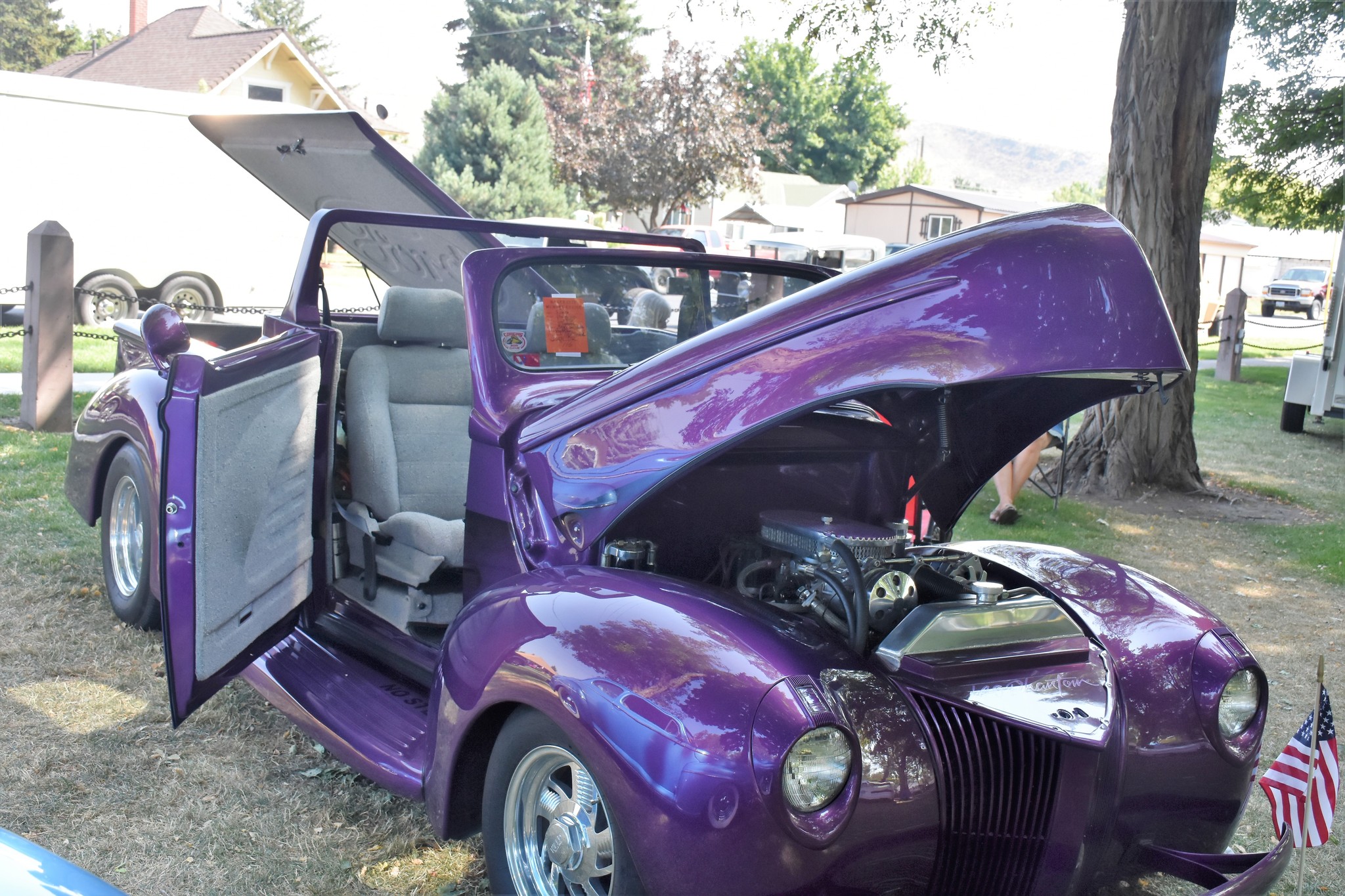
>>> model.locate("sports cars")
[60,107,1296,896]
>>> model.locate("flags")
[1258,683,1339,848]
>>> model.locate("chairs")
[1028,415,1071,511]
[523,302,618,368]
[346,285,474,623]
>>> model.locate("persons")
[989,421,1063,526]
[617,287,671,330]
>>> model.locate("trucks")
[0,67,389,327]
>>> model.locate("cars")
[491,216,912,301]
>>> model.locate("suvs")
[1260,265,1336,320]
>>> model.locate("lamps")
[848,180,858,197]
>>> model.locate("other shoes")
[998,504,1018,525]
[989,509,1023,521]
